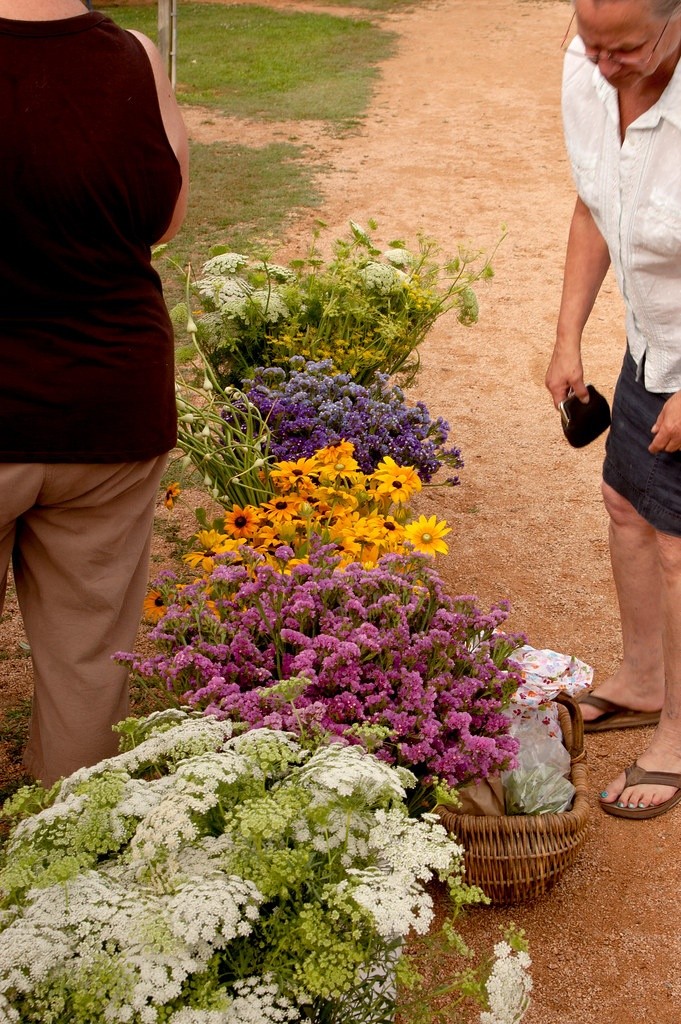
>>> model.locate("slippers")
[572,688,662,732]
[601,758,679,820]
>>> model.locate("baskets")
[436,690,591,905]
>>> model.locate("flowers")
[210,349,468,494]
[149,216,506,392]
[2,701,537,1023]
[167,370,278,514]
[108,544,530,800]
[135,443,449,623]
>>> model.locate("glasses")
[558,9,672,66]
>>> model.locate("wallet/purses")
[552,382,611,450]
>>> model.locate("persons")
[0,0,190,792]
[543,0,681,821]
